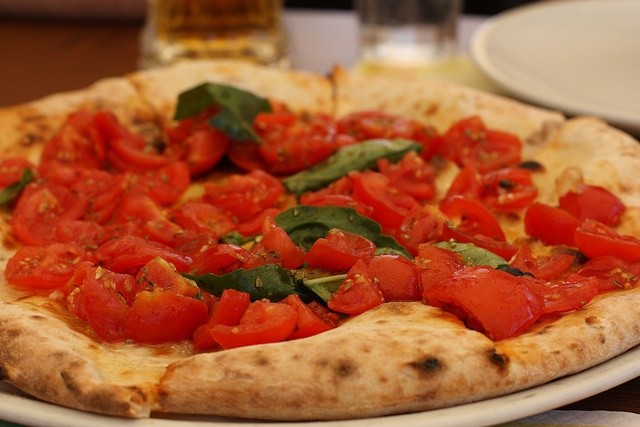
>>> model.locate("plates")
[469,1,639,132]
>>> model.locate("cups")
[354,0,458,62]
[139,0,288,65]
